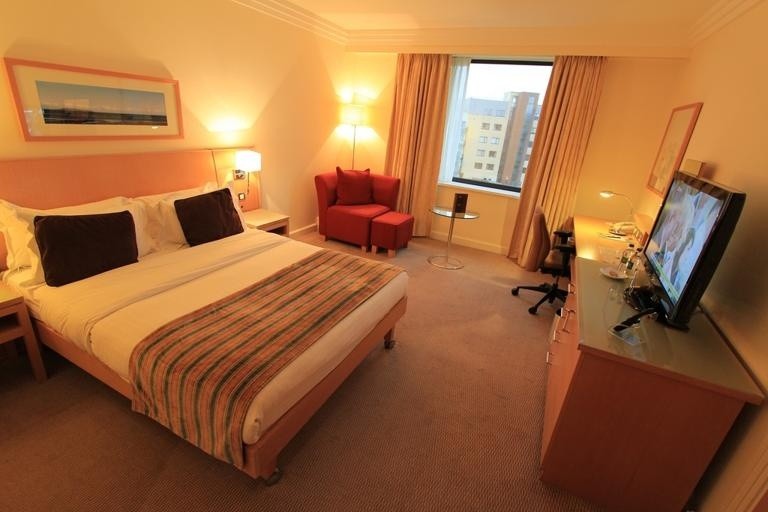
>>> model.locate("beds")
[0,142,417,489]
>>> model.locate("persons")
[653,182,724,305]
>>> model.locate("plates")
[600,265,629,281]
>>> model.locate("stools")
[369,207,416,261]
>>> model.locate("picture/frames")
[644,102,703,199]
[2,54,187,144]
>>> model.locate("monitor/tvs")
[624,170,746,329]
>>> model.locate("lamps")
[230,149,262,182]
[338,107,369,169]
[598,189,641,236]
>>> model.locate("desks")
[563,214,645,282]
[426,206,482,272]
[242,208,292,236]
[0,281,49,384]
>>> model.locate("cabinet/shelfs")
[540,255,766,511]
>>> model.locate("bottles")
[617,244,644,282]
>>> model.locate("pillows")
[135,180,214,239]
[158,181,249,249]
[29,209,140,290]
[172,189,245,248]
[332,164,374,207]
[0,193,158,270]
[10,199,160,291]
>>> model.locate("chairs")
[313,170,403,256]
[509,209,576,320]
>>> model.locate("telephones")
[612,222,634,234]
